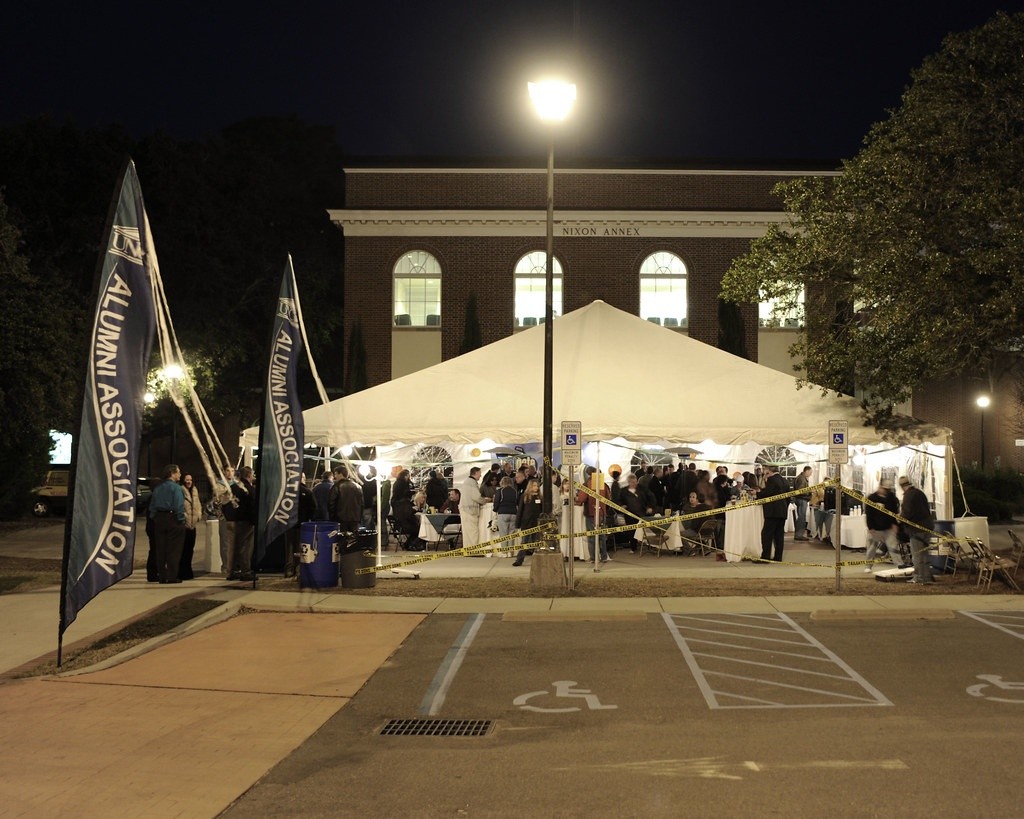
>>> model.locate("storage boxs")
[785,318,798,327]
[539,318,546,324]
[759,318,764,327]
[523,317,537,325]
[395,314,412,325]
[681,318,687,326]
[514,317,520,325]
[664,318,678,326]
[426,315,442,326]
[648,318,660,326]
[767,318,781,326]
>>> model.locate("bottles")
[853,506,858,516]
[858,505,861,516]
[849,508,853,516]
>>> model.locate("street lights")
[528,76,577,587]
[143,393,154,476]
[977,397,989,470]
[171,365,181,464]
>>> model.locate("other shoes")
[683,551,695,557]
[865,566,872,572]
[751,557,768,564]
[585,557,594,564]
[498,552,512,558]
[795,535,808,540]
[512,561,520,566]
[907,577,925,585]
[227,574,259,582]
[158,577,182,584]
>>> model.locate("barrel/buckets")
[953,516,990,569]
[928,520,956,575]
[300,521,341,588]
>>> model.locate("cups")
[429,506,434,514]
[664,509,671,518]
[731,500,736,505]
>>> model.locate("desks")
[477,502,520,558]
[784,502,869,550]
[560,505,589,562]
[416,513,450,550]
[724,499,775,564]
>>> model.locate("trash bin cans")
[340,528,377,590]
[298,519,340,591]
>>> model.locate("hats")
[755,464,762,470]
[880,480,894,490]
[899,476,912,486]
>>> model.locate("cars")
[30,469,70,516]
[136,478,162,509]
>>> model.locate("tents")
[236,299,977,573]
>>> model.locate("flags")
[60,160,157,636]
[253,259,305,550]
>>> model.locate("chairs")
[639,516,671,559]
[435,515,463,552]
[942,528,1024,594]
[385,515,410,552]
[689,519,717,558]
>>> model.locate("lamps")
[358,464,376,482]
[341,446,353,457]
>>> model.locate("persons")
[574,459,791,564]
[794,465,818,542]
[214,464,259,581]
[327,465,365,579]
[459,463,576,566]
[865,476,936,584]
[145,464,202,584]
[284,471,335,578]
[356,466,461,551]
